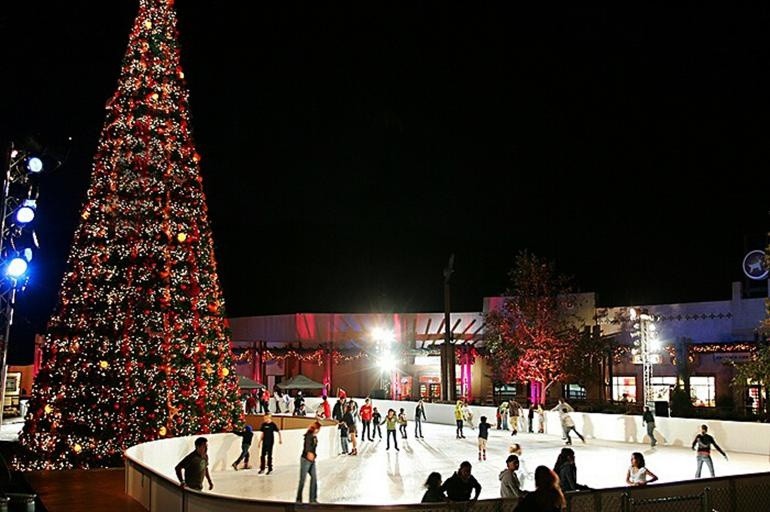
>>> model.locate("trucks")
[4,369,22,416]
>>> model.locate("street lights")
[369,322,400,399]
[0,140,43,429]
[629,307,663,417]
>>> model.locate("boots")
[455,434,466,439]
[496,425,586,445]
[338,433,423,455]
[478,454,487,462]
[232,462,273,475]
